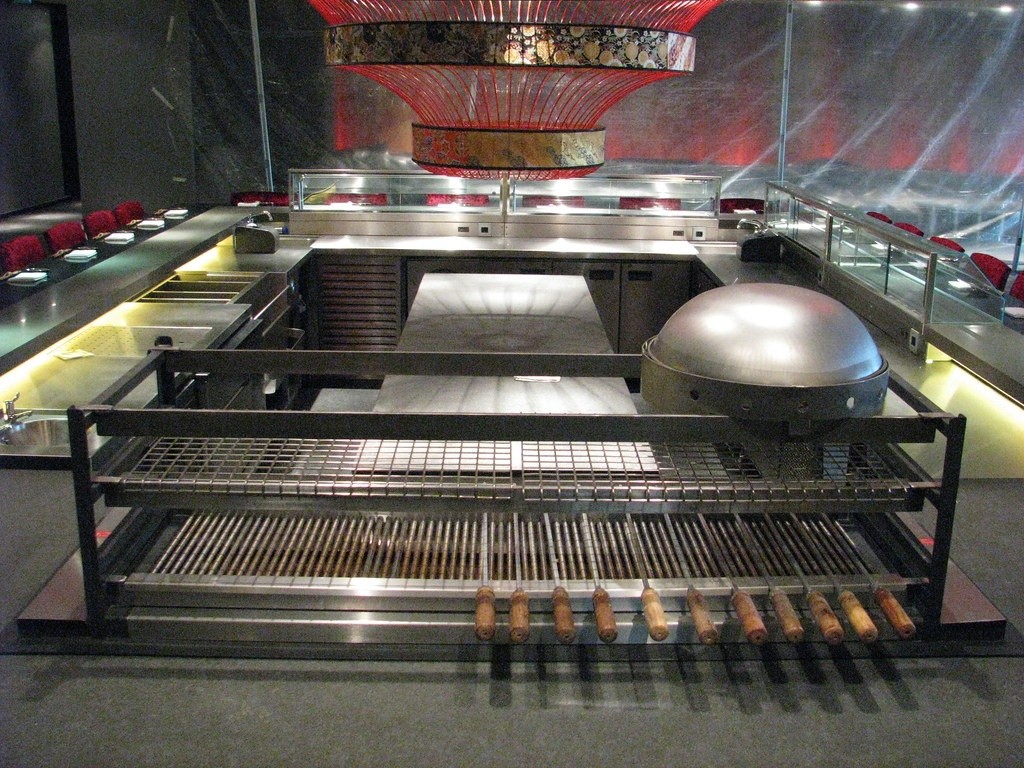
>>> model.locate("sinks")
[691,241,737,253]
[3,418,91,446]
[279,235,320,248]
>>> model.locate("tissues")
[234,223,280,253]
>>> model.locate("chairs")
[0,199,146,276]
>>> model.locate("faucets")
[2,392,33,423]
[736,218,767,233]
[248,211,273,221]
